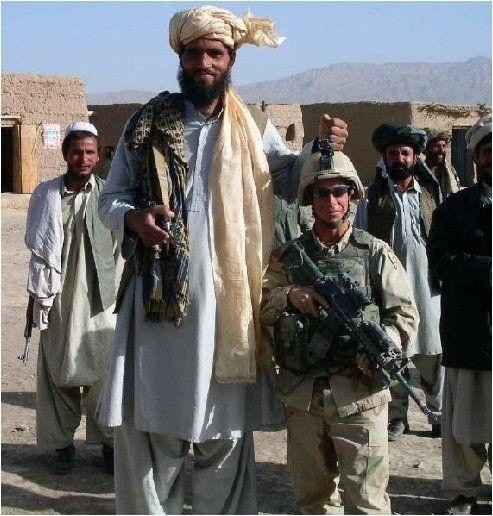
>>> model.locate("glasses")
[312,184,350,197]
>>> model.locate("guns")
[18,297,34,364]
[277,240,438,425]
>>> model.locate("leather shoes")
[387,419,408,440]
[431,424,441,436]
[102,445,115,475]
[446,496,477,514]
[46,441,75,474]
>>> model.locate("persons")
[98,6,349,515]
[424,128,460,203]
[24,123,121,475]
[275,194,311,248]
[258,150,421,515]
[426,113,492,514]
[352,124,443,441]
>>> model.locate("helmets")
[298,149,365,205]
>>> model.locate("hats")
[66,122,98,137]
